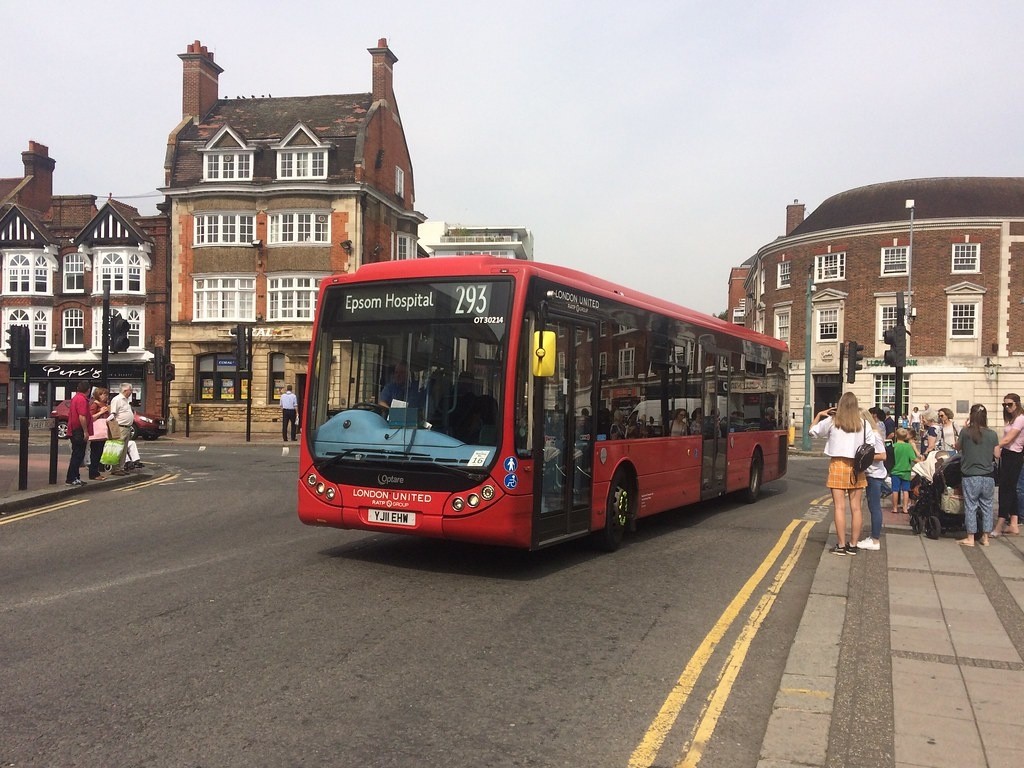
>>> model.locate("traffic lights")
[847,341,864,383]
[231,323,246,371]
[165,364,175,381]
[111,317,130,351]
[884,325,906,367]
[5,325,20,370]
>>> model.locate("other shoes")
[122,470,130,474]
[98,475,106,478]
[112,471,125,475]
[284,439,288,441]
[291,439,297,441]
[90,477,105,480]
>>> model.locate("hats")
[881,407,890,413]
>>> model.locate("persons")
[600,408,654,440]
[669,406,775,482]
[280,385,299,441]
[64,380,145,487]
[809,392,895,554]
[954,393,1024,546]
[891,402,961,547]
[378,360,424,410]
[453,371,478,442]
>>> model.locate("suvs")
[51,400,168,441]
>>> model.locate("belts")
[119,425,131,427]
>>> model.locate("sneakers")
[845,542,857,555]
[858,537,880,550]
[829,545,845,555]
[65,480,81,485]
[75,479,88,484]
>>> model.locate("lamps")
[907,308,917,325]
[340,240,352,250]
[251,239,263,248]
[747,292,754,299]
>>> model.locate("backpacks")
[850,419,875,485]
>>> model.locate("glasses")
[1002,402,1015,407]
[939,415,946,418]
[677,414,686,417]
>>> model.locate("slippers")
[989,531,1002,537]
[892,509,899,513]
[900,509,908,514]
[978,539,990,547]
[1002,527,1019,535]
[956,539,974,546]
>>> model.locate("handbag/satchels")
[88,418,108,440]
[941,487,964,514]
[72,428,86,444]
[295,416,299,425]
[100,439,125,465]
[109,421,121,439]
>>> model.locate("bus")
[298,255,788,554]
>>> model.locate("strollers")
[908,451,964,540]
